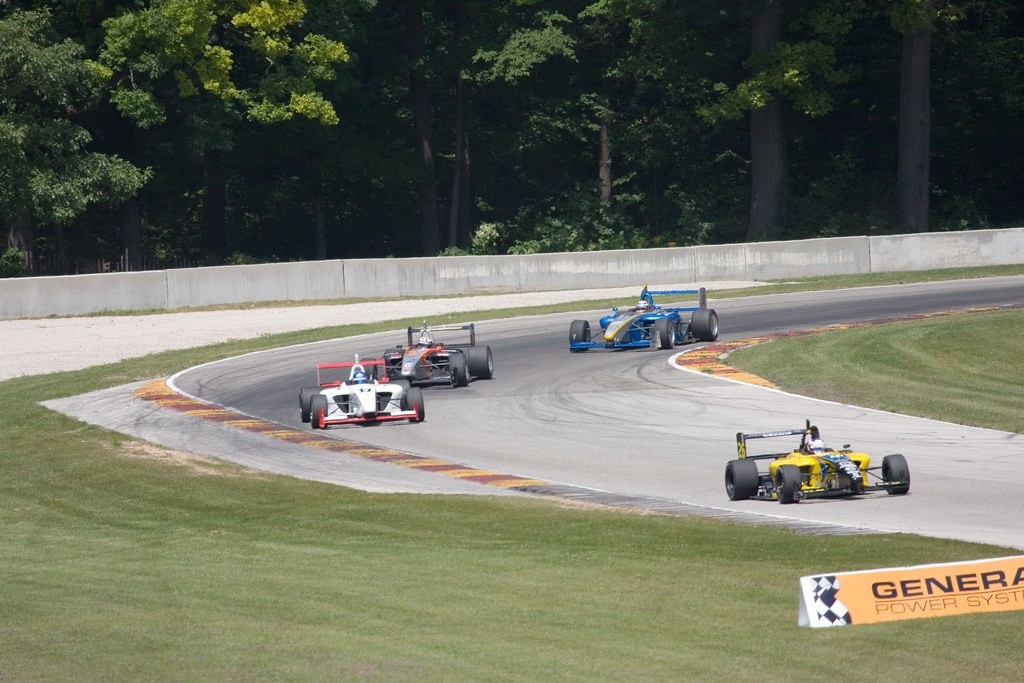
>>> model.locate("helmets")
[353,372,367,384]
[807,439,826,455]
[636,301,650,312]
[418,337,432,347]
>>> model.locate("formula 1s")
[299,353,425,430]
[725,419,910,504]
[382,319,494,389]
[569,284,719,353]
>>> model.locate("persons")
[806,439,825,453]
[636,301,649,312]
[418,337,432,348]
[352,371,368,384]
